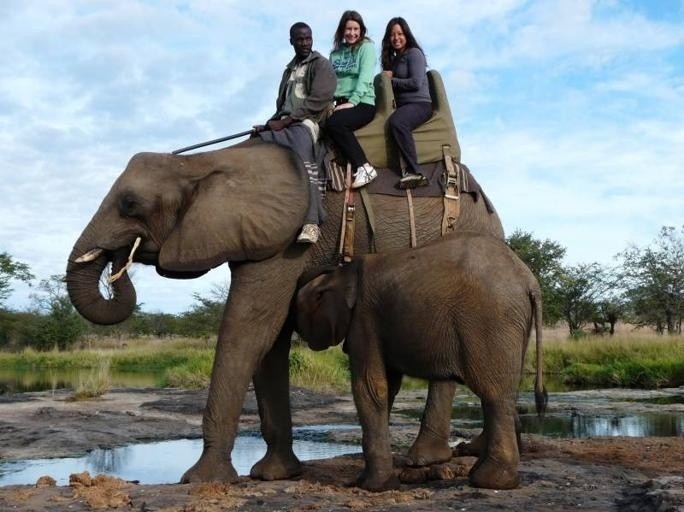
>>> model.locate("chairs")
[400,169,429,191]
[351,161,377,189]
[297,223,319,244]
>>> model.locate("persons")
[250,22,337,246]
[324,10,378,189]
[379,17,433,188]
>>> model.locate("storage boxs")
[355,65,462,168]
